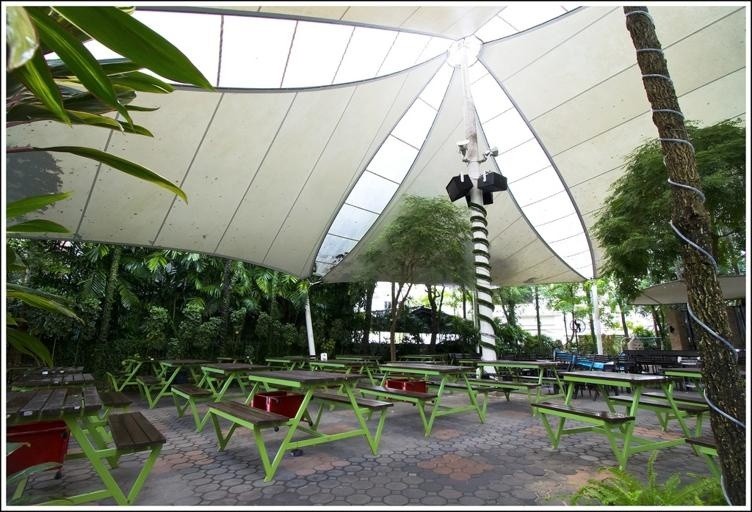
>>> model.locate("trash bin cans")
[167,372,188,384]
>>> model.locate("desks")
[7,365,130,506]
[196,364,264,435]
[379,363,485,437]
[245,369,377,483]
[552,372,700,475]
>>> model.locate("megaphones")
[485,145,499,156]
[456,140,470,156]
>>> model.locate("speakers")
[477,171,508,192]
[466,192,493,207]
[445,174,473,201]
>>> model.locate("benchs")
[530,401,635,472]
[94,378,134,416]
[205,400,290,484]
[94,355,703,388]
[303,390,395,455]
[354,383,436,439]
[641,391,708,406]
[466,379,541,417]
[429,380,496,424]
[105,412,166,505]
[608,395,710,438]
[171,385,214,431]
[684,434,715,485]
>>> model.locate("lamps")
[444,170,510,207]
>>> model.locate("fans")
[570,317,587,334]
